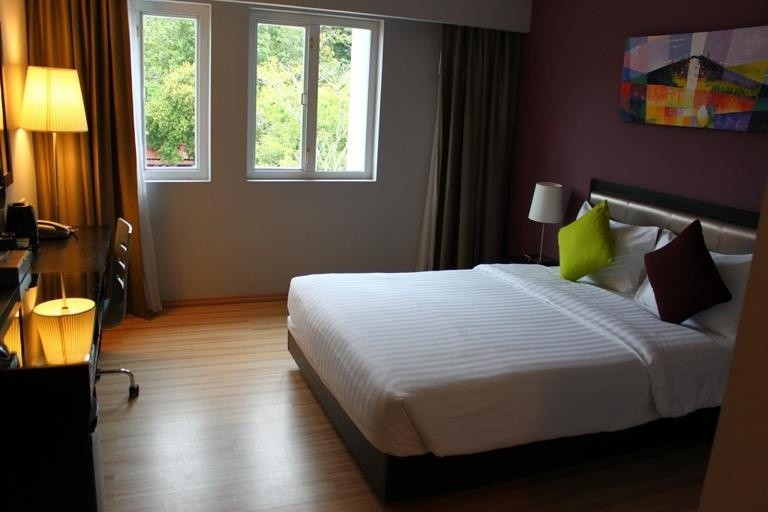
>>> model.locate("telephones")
[36,220,72,237]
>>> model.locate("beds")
[285,176,760,509]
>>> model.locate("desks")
[0,224,113,512]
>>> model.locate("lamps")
[527,181,565,263]
[32,271,96,368]
[17,65,89,223]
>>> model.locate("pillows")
[574,201,660,297]
[633,228,753,341]
[557,200,615,282]
[644,219,733,325]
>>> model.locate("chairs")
[95,217,139,398]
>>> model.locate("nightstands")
[507,253,559,266]
